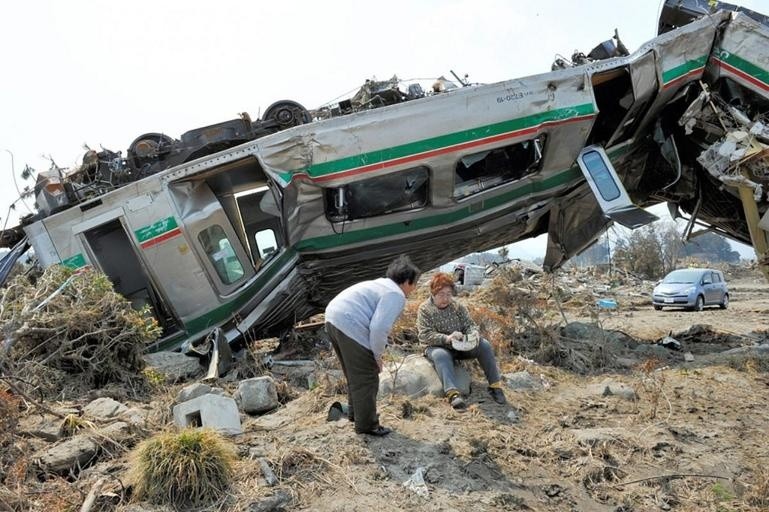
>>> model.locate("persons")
[322,251,423,438]
[415,270,507,409]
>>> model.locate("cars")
[651,268,730,311]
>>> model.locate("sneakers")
[450,391,466,409]
[355,422,391,436]
[485,385,506,407]
[347,410,380,421]
